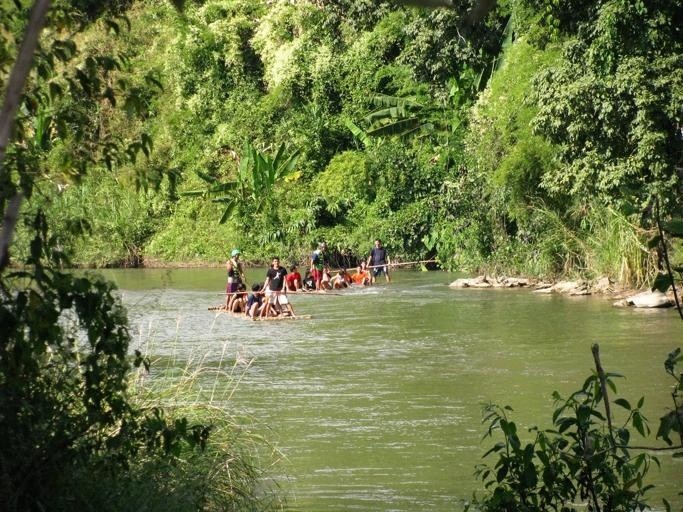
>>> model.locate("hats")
[231,249,240,257]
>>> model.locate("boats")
[208,304,312,322]
[280,283,398,296]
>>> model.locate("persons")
[223,238,391,319]
